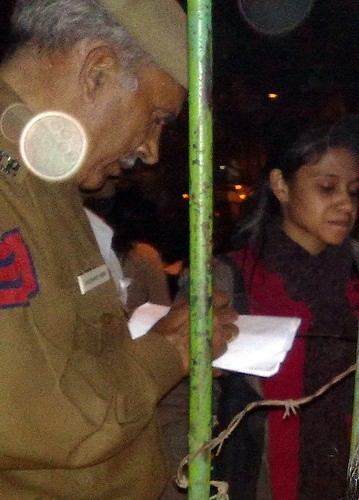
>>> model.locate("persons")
[175,109,359,500]
[107,186,171,327]
[0,1,241,500]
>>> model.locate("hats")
[103,1,189,94]
